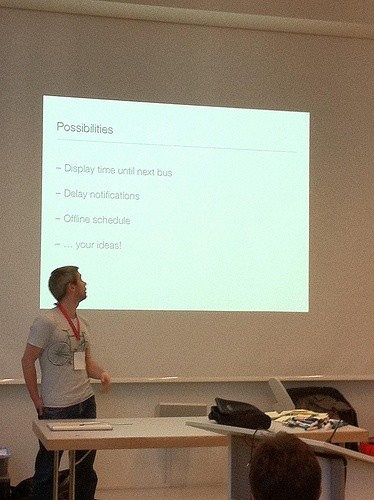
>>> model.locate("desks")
[32,413,369,500]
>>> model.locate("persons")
[250,430,323,500]
[22,265,112,500]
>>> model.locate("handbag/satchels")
[14,467,69,500]
[209,397,272,430]
